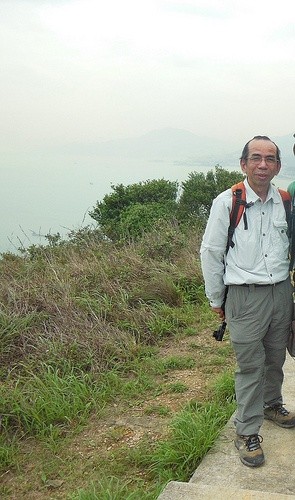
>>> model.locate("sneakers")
[264,396,295,428]
[234,433,265,466]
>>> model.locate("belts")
[237,277,289,287]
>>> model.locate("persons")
[200,135,295,466]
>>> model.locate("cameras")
[213,322,226,341]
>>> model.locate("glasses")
[247,155,280,166]
